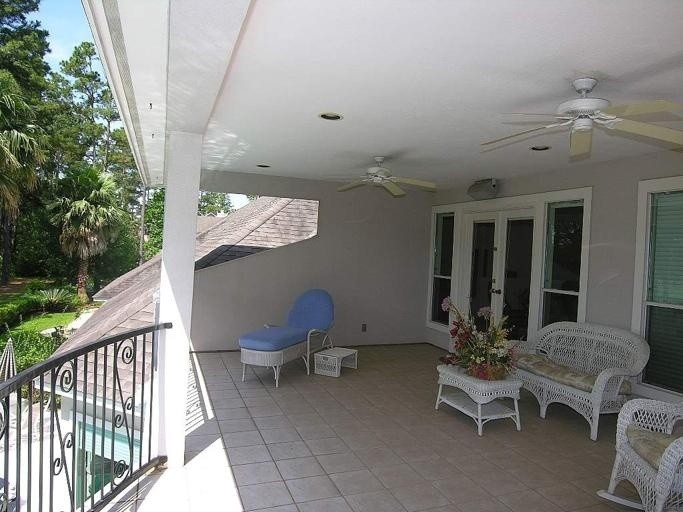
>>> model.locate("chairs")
[595,396,682,512]
[263,287,336,378]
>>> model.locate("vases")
[464,359,506,381]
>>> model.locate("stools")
[237,324,311,387]
[312,346,359,377]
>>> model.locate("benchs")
[496,318,652,443]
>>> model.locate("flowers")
[433,292,525,382]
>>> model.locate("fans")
[310,154,438,199]
[476,76,683,165]
